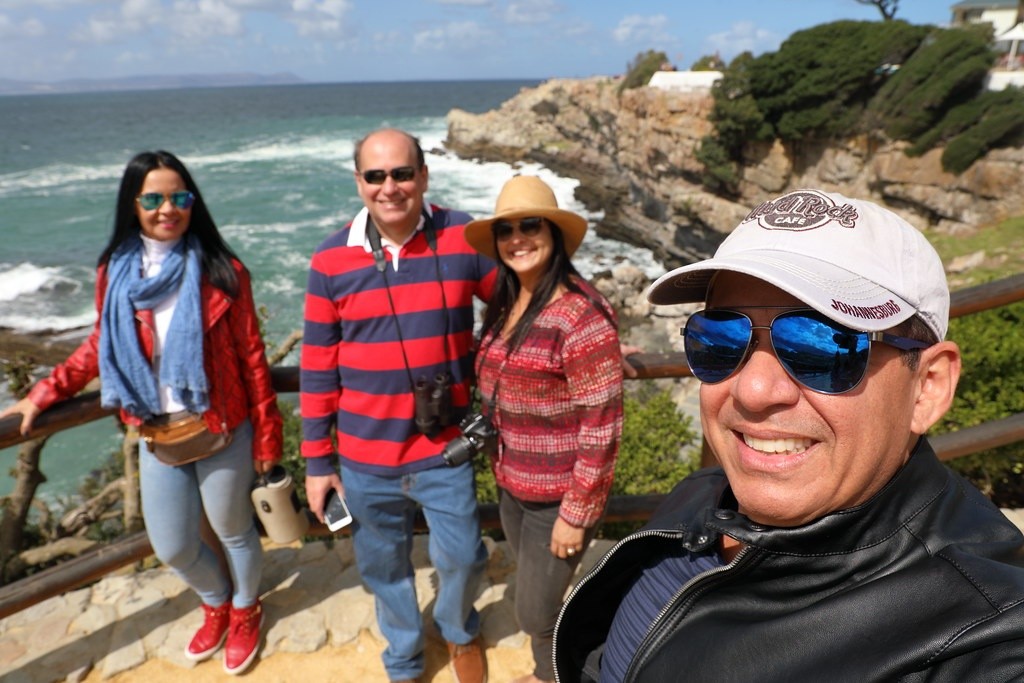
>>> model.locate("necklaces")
[511,296,530,316]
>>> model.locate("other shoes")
[390,676,421,683]
[435,619,488,683]
[515,674,556,683]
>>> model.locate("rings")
[568,549,576,554]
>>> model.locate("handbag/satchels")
[139,405,236,466]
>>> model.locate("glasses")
[679,304,932,396]
[132,191,198,211]
[491,216,544,239]
[355,166,423,187]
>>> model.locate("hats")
[644,188,951,345]
[462,173,589,263]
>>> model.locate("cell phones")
[323,487,353,532]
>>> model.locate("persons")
[463,176,624,683]
[552,190,1024,683]
[0,151,286,676]
[300,130,496,683]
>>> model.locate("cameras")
[440,412,497,466]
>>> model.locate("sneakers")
[184,597,232,662]
[224,600,267,676]
